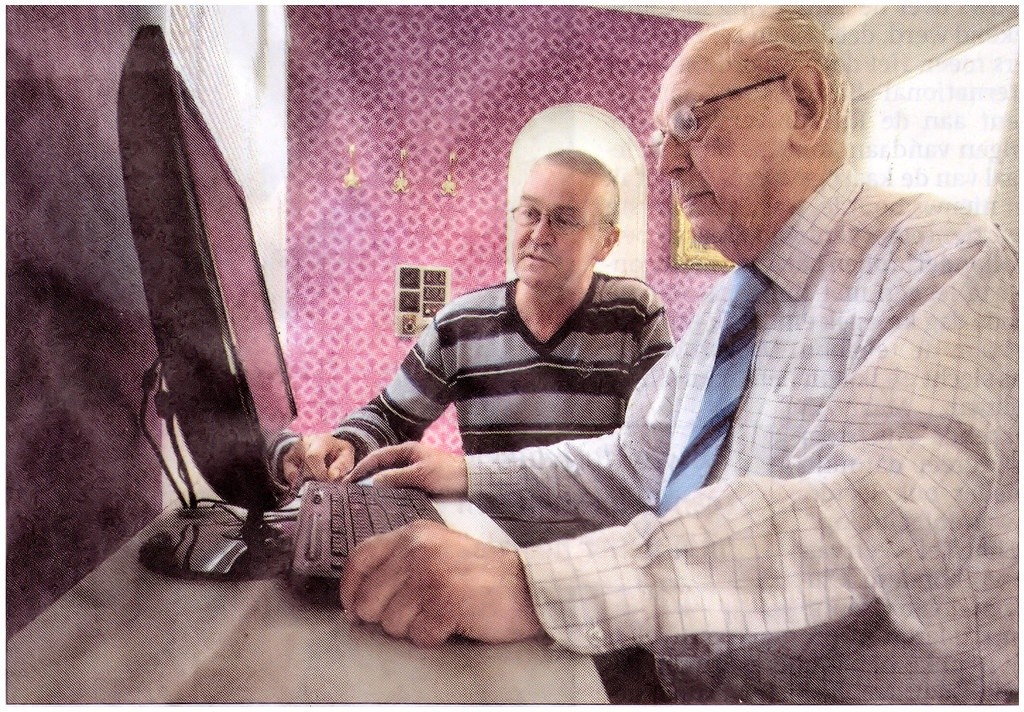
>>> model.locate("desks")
[7,490,614,705]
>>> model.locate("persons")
[285,149,674,705]
[341,4,1019,704]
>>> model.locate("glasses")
[511,205,613,235]
[646,73,787,149]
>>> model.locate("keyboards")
[291,481,449,591]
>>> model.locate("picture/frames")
[670,180,738,272]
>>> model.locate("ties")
[658,267,772,507]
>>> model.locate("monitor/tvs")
[116,25,297,581]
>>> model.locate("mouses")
[356,467,404,487]
